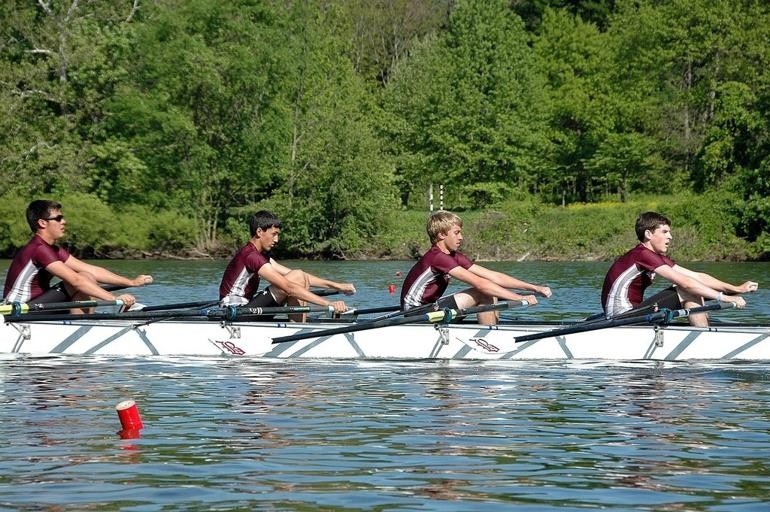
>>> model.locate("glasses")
[45,215,63,222]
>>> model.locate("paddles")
[117,285,345,310]
[209,299,528,358]
[458,300,738,354]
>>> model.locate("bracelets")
[716,291,723,304]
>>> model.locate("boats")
[2,307,770,365]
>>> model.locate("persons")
[219,210,357,324]
[399,210,553,326]
[2,199,154,316]
[600,210,760,328]
